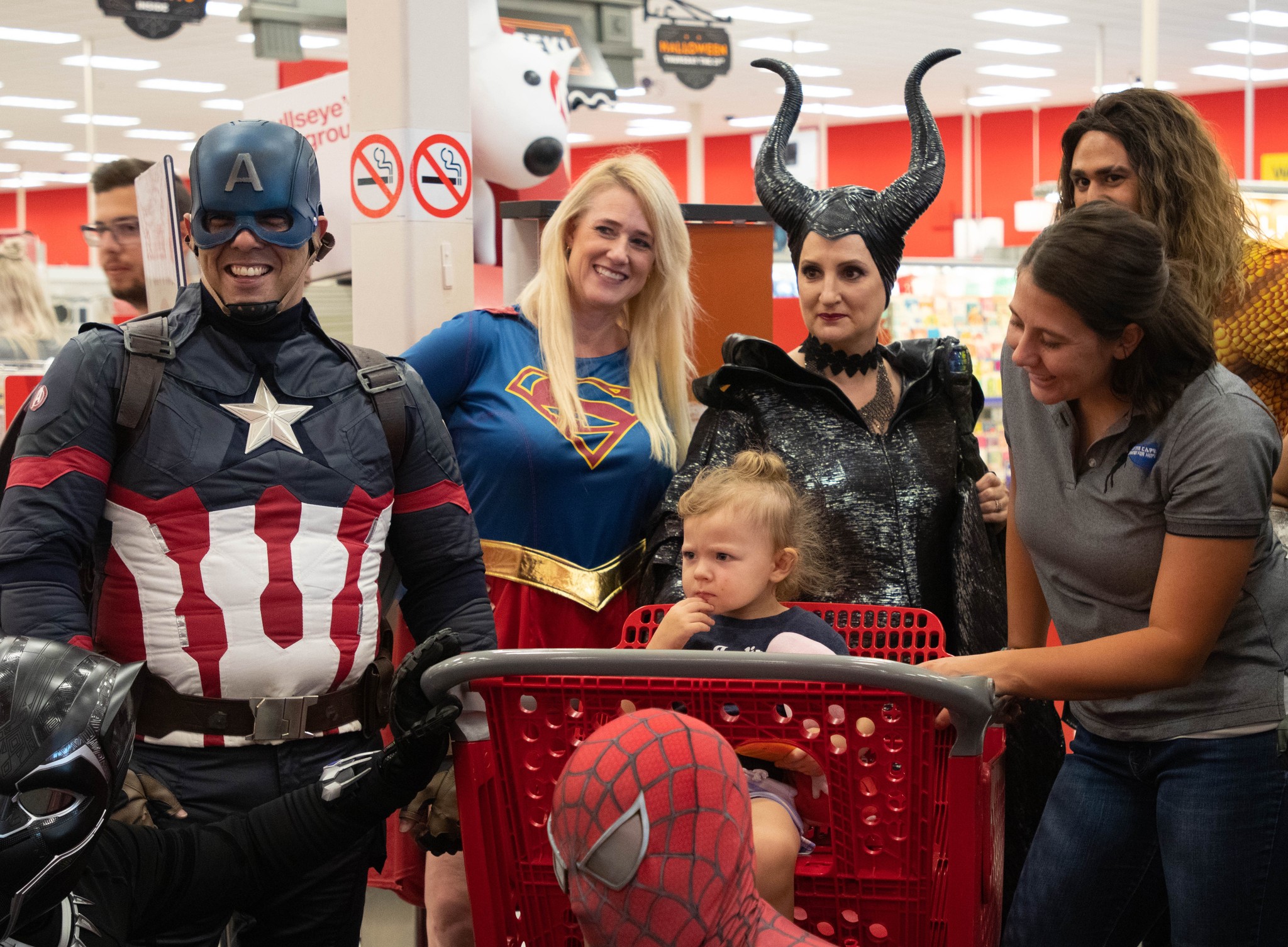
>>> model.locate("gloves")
[371,628,463,811]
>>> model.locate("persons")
[383,152,703,947]
[634,449,853,924]
[968,89,1288,557]
[545,710,839,947]
[88,159,194,328]
[914,199,1288,947]
[645,47,1066,918]
[0,237,67,363]
[0,118,501,947]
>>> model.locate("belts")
[135,680,368,740]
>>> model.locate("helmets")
[186,119,322,249]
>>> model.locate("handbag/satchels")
[929,334,1012,655]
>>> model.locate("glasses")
[79,222,142,247]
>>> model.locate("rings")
[993,498,1004,513]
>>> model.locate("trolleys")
[421,600,1019,947]
